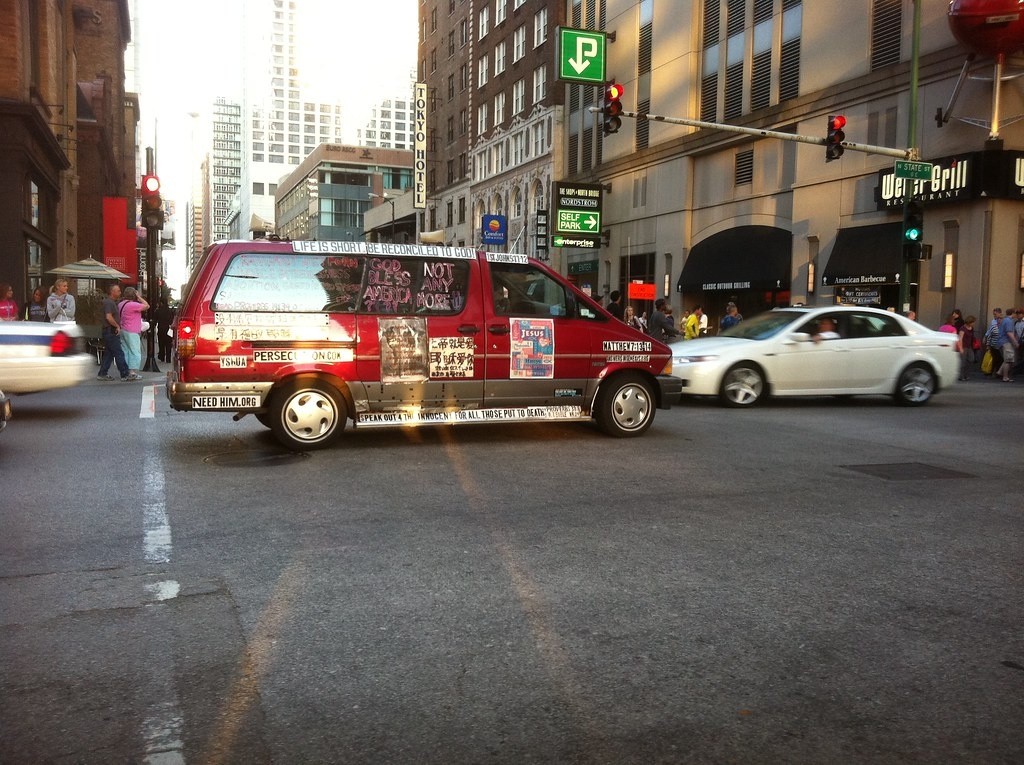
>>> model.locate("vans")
[166,239,688,453]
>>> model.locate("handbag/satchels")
[981,350,993,374]
[972,336,981,350]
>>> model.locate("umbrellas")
[44,255,131,299]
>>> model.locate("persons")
[607,290,622,321]
[46,279,76,322]
[812,317,841,341]
[153,296,174,363]
[624,306,643,332]
[591,294,604,306]
[96,285,137,381]
[21,285,50,322]
[905,311,916,320]
[720,303,743,330]
[952,307,1024,382]
[638,311,647,329]
[680,304,708,340]
[663,304,675,337]
[0,283,19,321]
[938,314,957,334]
[117,287,150,380]
[648,299,684,343]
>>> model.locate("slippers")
[1003,379,1015,382]
[995,373,1002,379]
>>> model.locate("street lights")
[368,192,395,244]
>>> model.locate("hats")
[592,294,604,302]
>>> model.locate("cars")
[0,390,13,434]
[659,304,962,409]
[0,316,98,396]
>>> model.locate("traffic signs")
[556,209,600,234]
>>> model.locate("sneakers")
[97,374,114,381]
[121,374,137,380]
[133,372,142,380]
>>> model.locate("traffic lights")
[904,201,923,242]
[826,115,847,162]
[141,175,164,230]
[603,82,624,134]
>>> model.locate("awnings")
[677,226,791,291]
[821,221,917,286]
